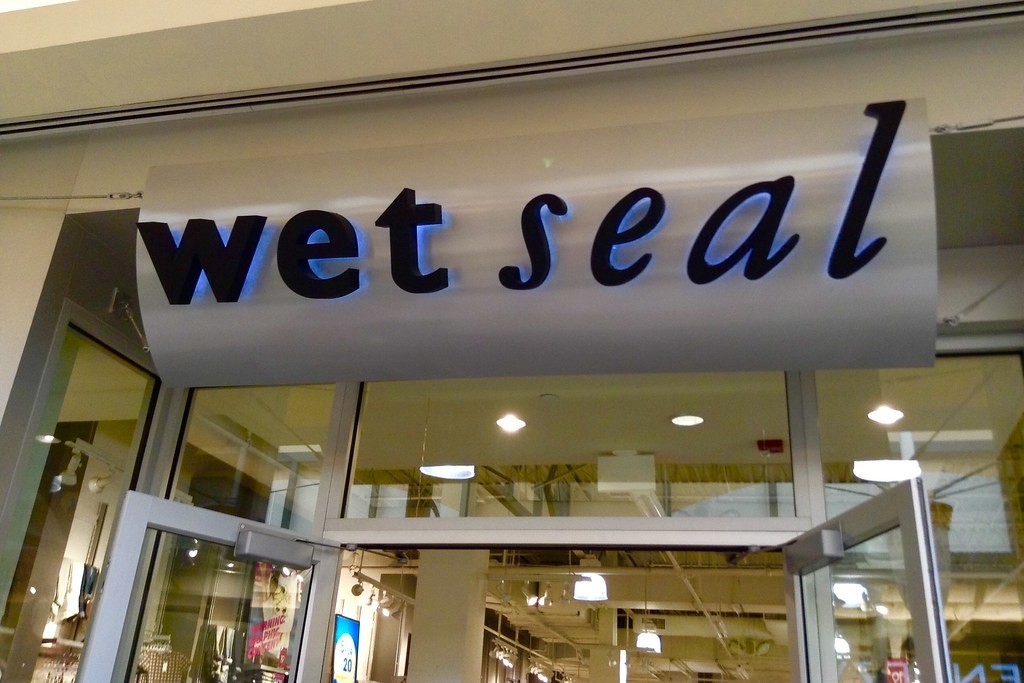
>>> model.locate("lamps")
[489,646,517,668]
[574,554,608,600]
[833,592,845,609]
[860,592,874,613]
[521,587,538,607]
[382,603,398,616]
[538,668,554,683]
[538,596,552,608]
[352,584,364,596]
[636,621,661,653]
